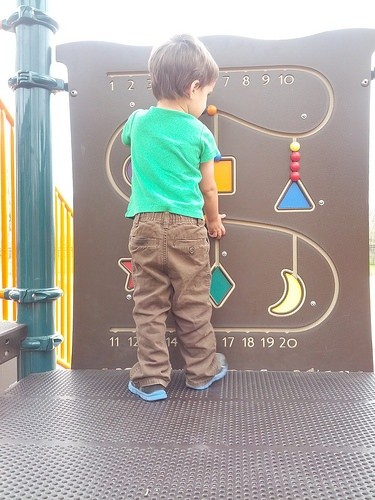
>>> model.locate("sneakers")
[128,380,168,401]
[186,353,228,390]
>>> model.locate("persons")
[121,33,227,401]
